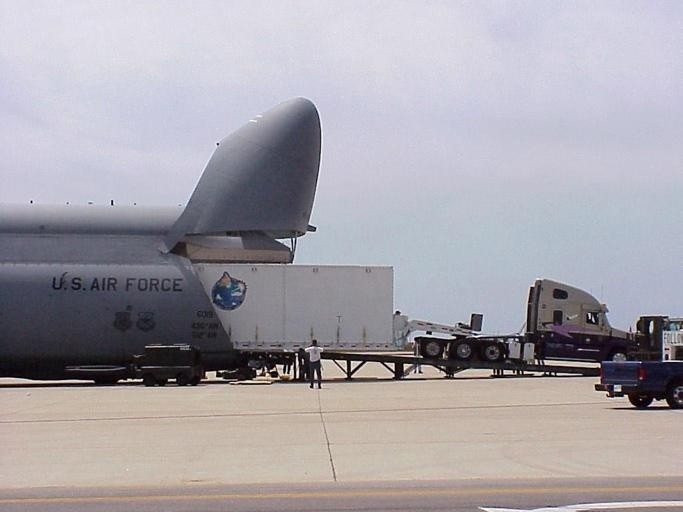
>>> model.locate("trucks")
[414,278,635,363]
[595,359,683,409]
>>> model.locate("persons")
[413,364,425,374]
[298,347,316,380]
[304,339,323,389]
